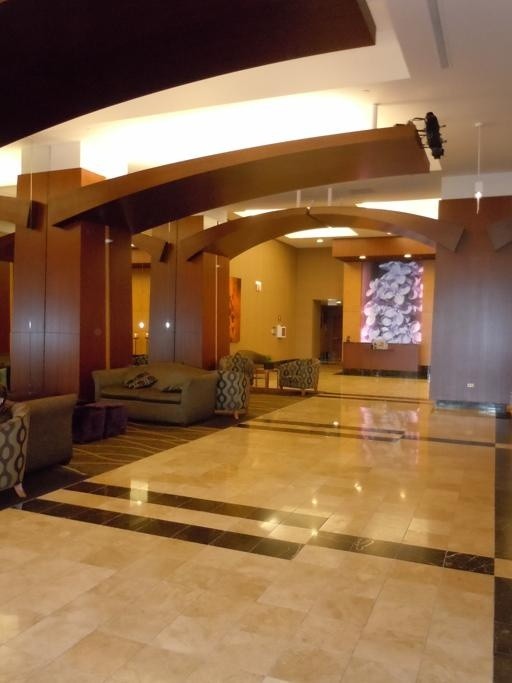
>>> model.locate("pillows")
[162,385,182,392]
[120,368,158,390]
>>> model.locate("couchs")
[90,363,219,427]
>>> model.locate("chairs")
[212,370,251,419]
[135,356,146,365]
[20,394,82,470]
[78,402,128,437]
[277,357,320,396]
[0,403,31,498]
[219,354,255,392]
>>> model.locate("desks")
[343,342,418,377]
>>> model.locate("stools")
[73,404,106,443]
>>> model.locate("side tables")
[254,369,269,388]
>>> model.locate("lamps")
[133,332,138,355]
[475,121,485,215]
[145,332,149,354]
